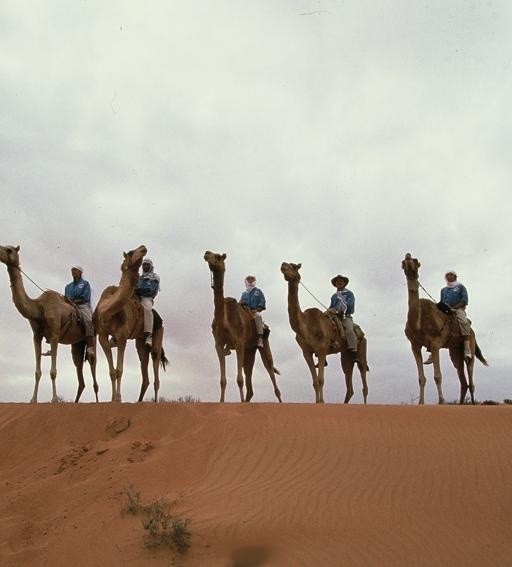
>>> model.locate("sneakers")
[315,362,327,367]
[41,351,52,356]
[424,355,433,364]
[465,348,472,359]
[257,339,263,348]
[224,350,231,355]
[86,347,94,355]
[145,337,153,347]
[352,352,360,362]
[110,337,117,347]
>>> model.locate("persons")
[38,265,95,359]
[315,275,360,368]
[223,274,266,355]
[423,271,472,365]
[110,258,161,347]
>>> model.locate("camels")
[402,253,489,405]
[204,251,282,403]
[0,245,98,403]
[93,245,170,403]
[280,262,369,404]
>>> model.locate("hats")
[244,274,256,281]
[445,270,457,280]
[142,259,153,266]
[331,274,349,287]
[71,265,83,273]
